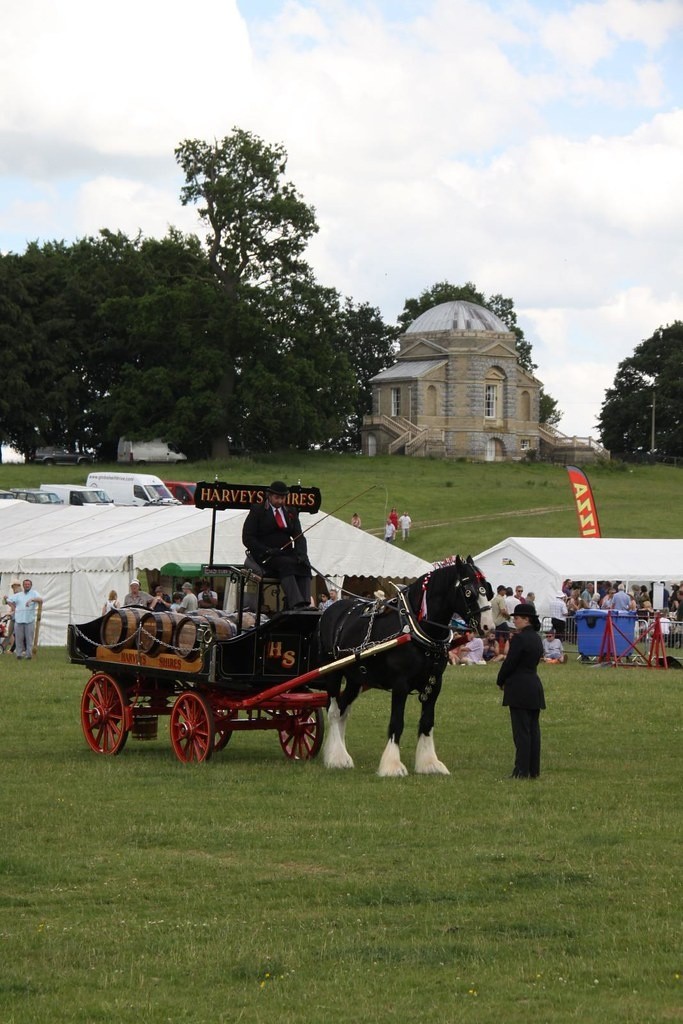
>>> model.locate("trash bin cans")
[572,608,639,662]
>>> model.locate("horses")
[314,552,498,778]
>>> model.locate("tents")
[0,498,438,647]
[471,537,683,628]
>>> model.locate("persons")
[318,589,388,613]
[350,513,361,527]
[102,580,219,617]
[388,505,399,539]
[384,520,397,543]
[450,585,536,666]
[495,604,546,780]
[242,480,320,612]
[4,578,42,660]
[399,512,411,542]
[540,577,683,664]
